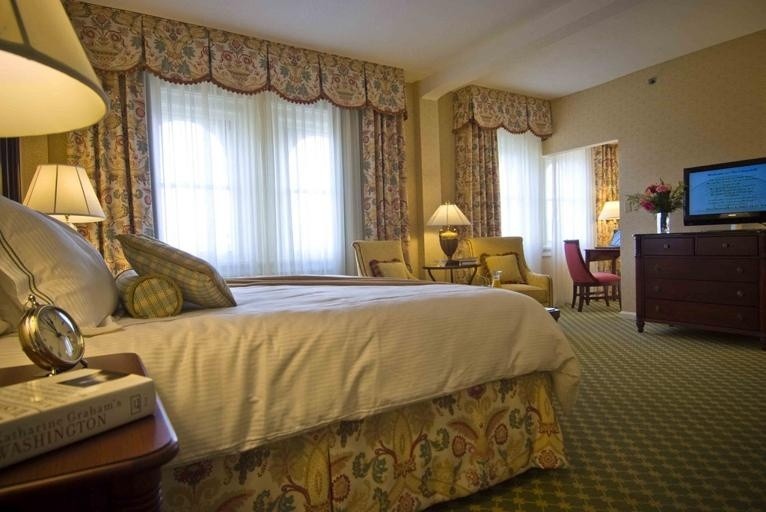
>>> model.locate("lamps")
[423,200,473,266]
[598,200,620,246]
[22,163,106,223]
[0,0,110,138]
[114,231,236,309]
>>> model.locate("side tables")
[423,264,481,285]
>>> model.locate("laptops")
[595,229,620,249]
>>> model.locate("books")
[0,367,160,472]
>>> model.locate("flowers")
[623,176,688,229]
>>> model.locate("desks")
[585,248,620,304]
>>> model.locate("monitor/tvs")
[683,157,766,230]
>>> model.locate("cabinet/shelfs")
[633,229,766,351]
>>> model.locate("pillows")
[480,252,527,284]
[0,192,121,335]
[115,268,183,318]
[369,259,409,279]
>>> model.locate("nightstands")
[0,353,179,512]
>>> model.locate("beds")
[0,273,579,512]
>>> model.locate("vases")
[657,212,672,233]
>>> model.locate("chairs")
[465,236,553,308]
[565,239,621,313]
[352,239,420,280]
